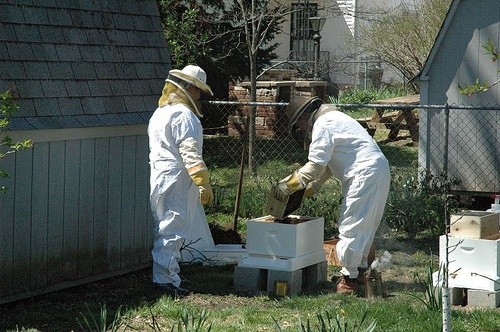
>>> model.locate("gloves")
[302,182,314,201]
[273,170,304,202]
[186,161,214,205]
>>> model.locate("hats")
[286,96,324,133]
[169,65,213,96]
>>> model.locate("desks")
[366,95,420,142]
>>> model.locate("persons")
[271,95,391,299]
[146,64,218,297]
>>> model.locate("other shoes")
[153,283,189,298]
[320,273,360,296]
[331,267,367,298]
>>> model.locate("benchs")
[356,113,399,137]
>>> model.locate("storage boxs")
[440,233,500,277]
[451,210,500,238]
[246,214,325,258]
[265,183,307,219]
